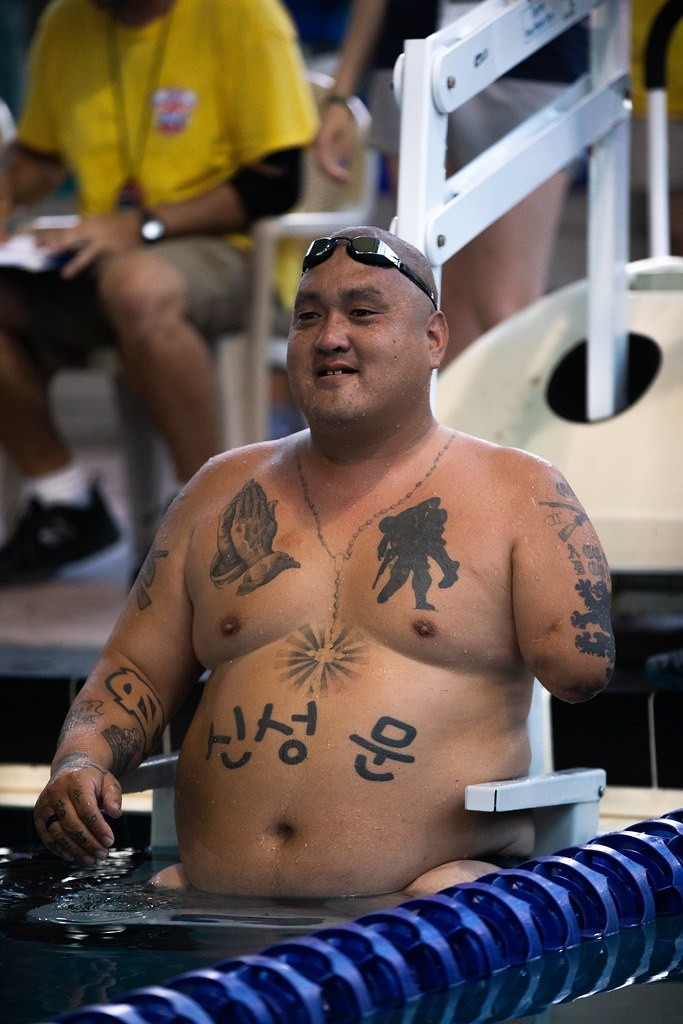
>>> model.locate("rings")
[44,815,59,829]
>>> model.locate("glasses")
[301,234,438,312]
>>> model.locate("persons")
[316,1,598,353]
[30,219,616,902]
[0,1,317,590]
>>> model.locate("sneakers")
[0,467,125,592]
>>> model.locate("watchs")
[140,210,165,246]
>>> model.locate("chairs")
[96,65,374,558]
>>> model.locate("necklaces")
[102,10,173,211]
